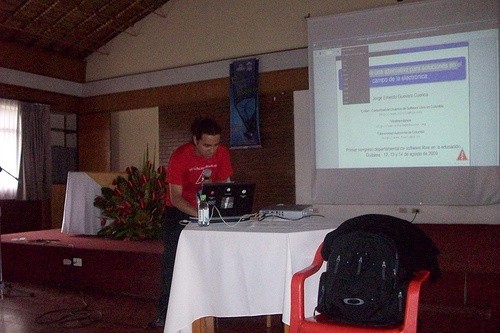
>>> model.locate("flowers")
[93,142,168,241]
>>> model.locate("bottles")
[198,194,209,227]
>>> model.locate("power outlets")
[412,208,421,214]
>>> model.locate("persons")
[159,118,235,327]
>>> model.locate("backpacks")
[313,230,405,327]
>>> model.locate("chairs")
[289,213,441,333]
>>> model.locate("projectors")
[258,203,314,220]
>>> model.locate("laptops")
[188,181,257,223]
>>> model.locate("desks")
[60,171,130,236]
[163,217,340,333]
